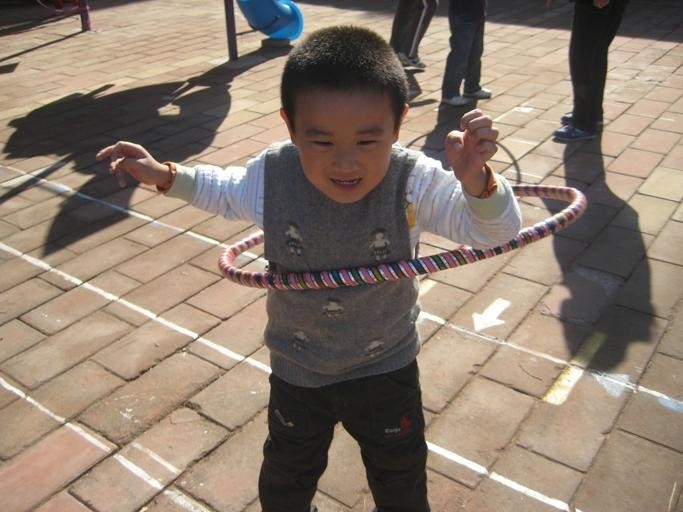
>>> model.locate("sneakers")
[552,122,602,142]
[463,87,492,99]
[560,112,604,126]
[441,95,474,107]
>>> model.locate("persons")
[386,0,439,73]
[440,0,492,106]
[551,0,629,142]
[95,25,521,512]
